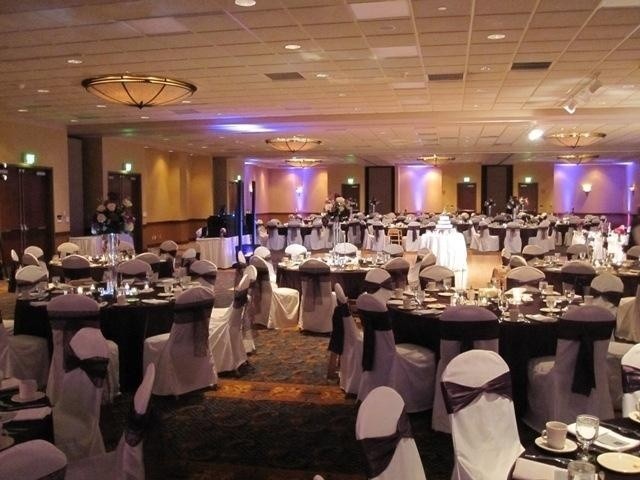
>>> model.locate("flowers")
[324,193,353,221]
[485,197,495,206]
[92,196,136,236]
[370,197,379,205]
[506,195,529,209]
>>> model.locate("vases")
[512,208,517,220]
[372,204,375,214]
[488,205,491,216]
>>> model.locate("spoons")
[598,419,632,435]
[526,453,572,466]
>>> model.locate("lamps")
[555,73,602,114]
[546,132,606,148]
[556,153,599,167]
[284,159,321,167]
[417,155,456,168]
[81,74,197,107]
[265,136,322,153]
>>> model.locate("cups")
[75,283,153,295]
[18,379,37,398]
[584,295,593,304]
[330,250,390,270]
[542,421,566,448]
[567,460,595,479]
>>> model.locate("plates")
[567,419,640,453]
[598,451,640,475]
[142,299,168,304]
[580,303,598,306]
[385,282,447,310]
[438,293,453,296]
[536,436,577,453]
[12,392,44,403]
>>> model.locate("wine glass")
[577,415,597,463]
[540,281,575,316]
[498,299,508,319]
[415,290,425,310]
[547,251,615,267]
